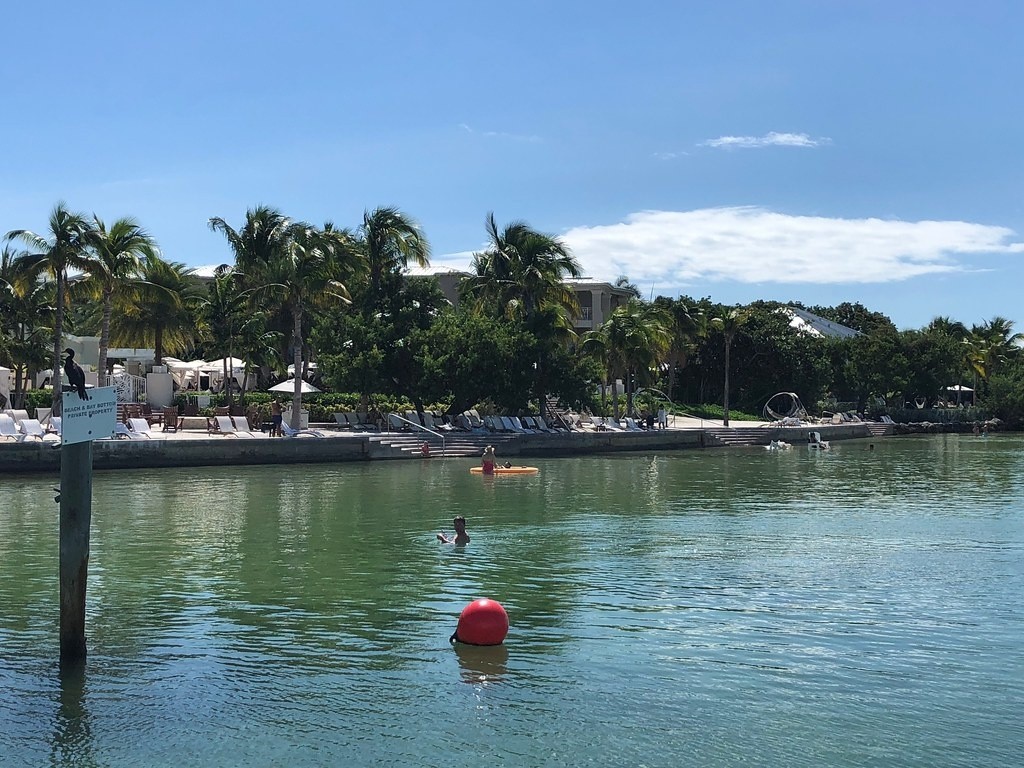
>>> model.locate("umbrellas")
[268,378,322,393]
[940,385,974,391]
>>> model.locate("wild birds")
[60,347,89,402]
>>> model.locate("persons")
[421,440,429,457]
[600,416,611,429]
[992,415,1000,423]
[272,397,287,437]
[972,422,979,436]
[206,380,222,393]
[646,401,655,429]
[657,404,666,429]
[481,446,497,474]
[436,515,470,544]
[549,413,583,430]
[981,425,988,437]
[795,409,813,422]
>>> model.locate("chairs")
[206,404,267,438]
[0,402,185,442]
[332,413,377,433]
[881,416,896,424]
[820,412,861,425]
[279,419,325,437]
[380,411,646,434]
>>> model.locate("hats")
[485,445,494,453]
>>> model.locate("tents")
[162,357,249,393]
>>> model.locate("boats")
[808,431,832,452]
[469,461,539,474]
